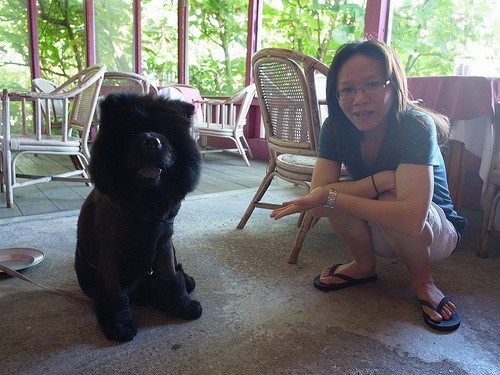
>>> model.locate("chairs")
[194,83,257,166]
[237,47,351,263]
[31,76,76,157]
[0,47,106,208]
[93,71,159,136]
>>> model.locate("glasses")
[335,80,390,100]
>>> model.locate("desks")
[100,84,202,145]
[404,75,500,259]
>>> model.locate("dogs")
[74,94,203,342]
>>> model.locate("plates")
[0,248,45,272]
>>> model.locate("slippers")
[313,264,377,291]
[418,296,460,331]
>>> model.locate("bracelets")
[371,175,379,194]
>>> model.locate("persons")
[269,40,466,331]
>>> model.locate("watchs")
[324,189,337,208]
